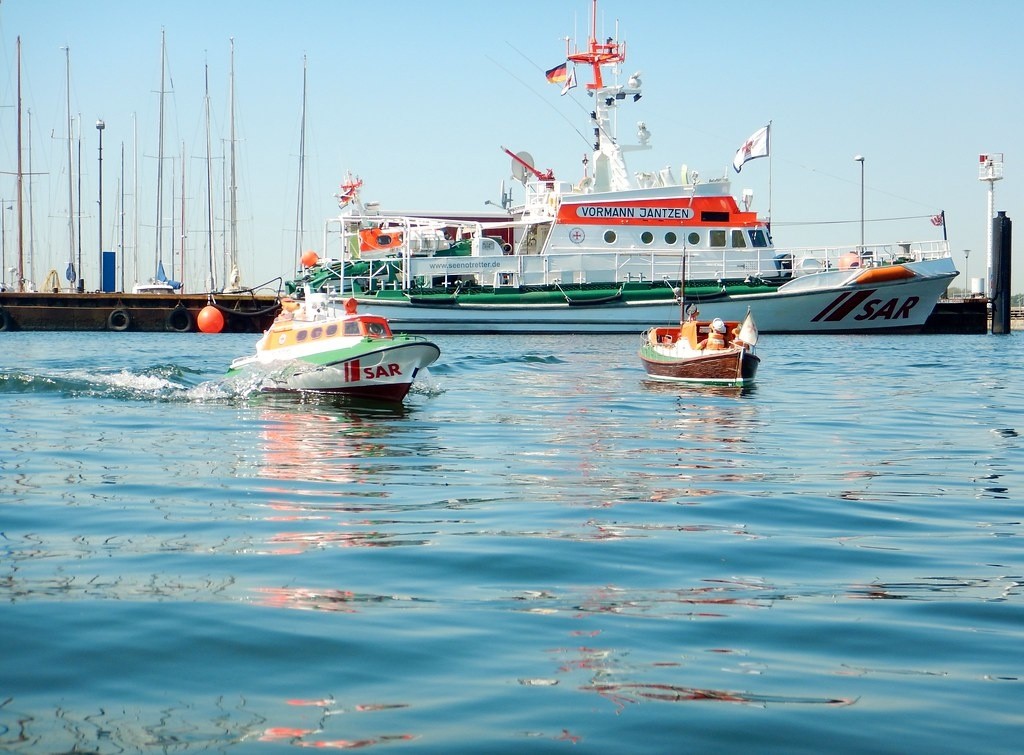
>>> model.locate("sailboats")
[0,29,304,332]
[638,233,761,387]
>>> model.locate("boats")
[283,0,960,334]
[222,280,441,401]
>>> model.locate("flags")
[545,63,566,83]
[733,125,770,173]
[739,310,759,347]
[561,67,577,96]
[930,213,943,226]
[337,188,353,210]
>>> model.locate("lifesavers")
[109,308,131,332]
[165,306,193,333]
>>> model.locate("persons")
[697,318,729,351]
[729,324,749,350]
